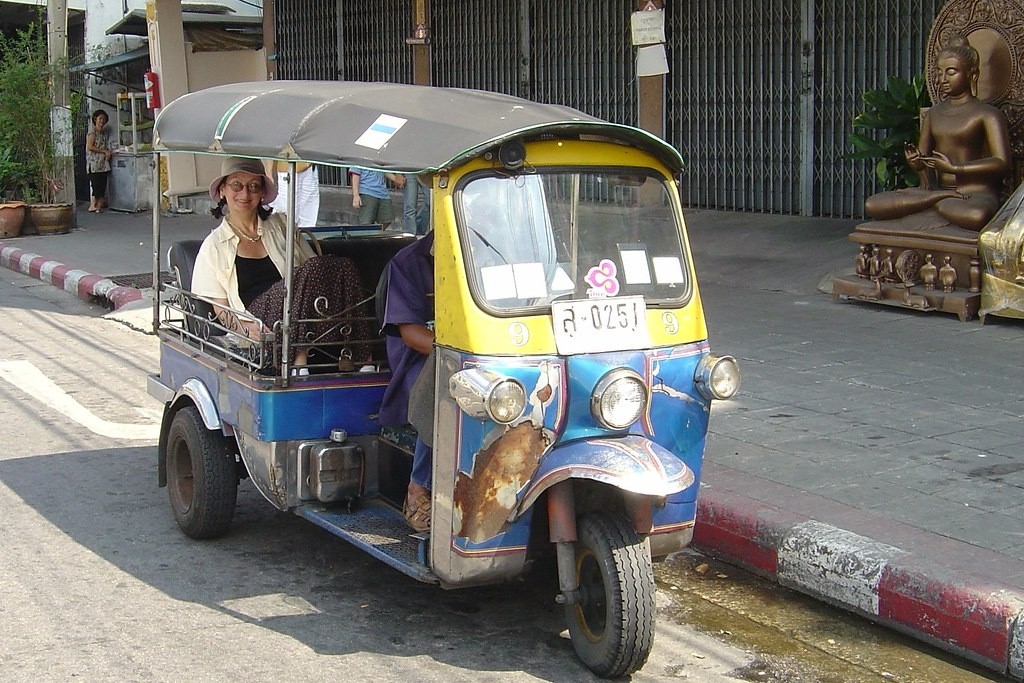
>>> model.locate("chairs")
[377,259,417,514]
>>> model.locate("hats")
[209,157,277,205]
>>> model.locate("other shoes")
[88,208,99,212]
[359,365,379,373]
[292,368,310,376]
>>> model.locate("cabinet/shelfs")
[116,93,155,155]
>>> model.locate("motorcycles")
[149,80,741,681]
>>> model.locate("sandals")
[403,494,431,532]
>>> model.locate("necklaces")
[227,220,261,242]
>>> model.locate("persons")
[190,156,378,376]
[395,173,430,236]
[84,109,113,213]
[939,255,958,286]
[378,223,437,534]
[879,247,898,279]
[349,167,406,231]
[856,244,869,275]
[866,247,882,276]
[266,147,320,228]
[919,253,939,284]
[863,34,1010,232]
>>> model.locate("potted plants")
[0,6,88,238]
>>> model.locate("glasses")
[224,181,262,193]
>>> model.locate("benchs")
[166,234,418,372]
